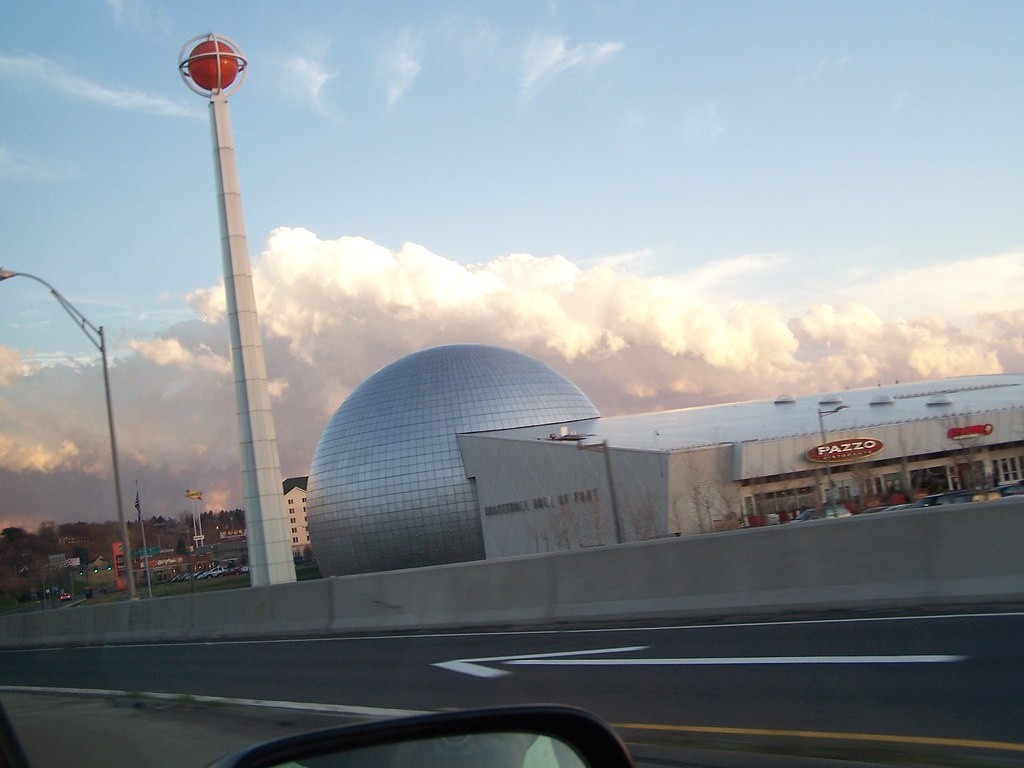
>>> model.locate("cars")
[789,505,853,524]
[863,479,1024,515]
[168,564,249,583]
[60,592,72,601]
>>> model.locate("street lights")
[0,267,136,602]
[816,405,850,517]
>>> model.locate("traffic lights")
[107,567,112,571]
[79,572,83,576]
[94,569,98,573]
